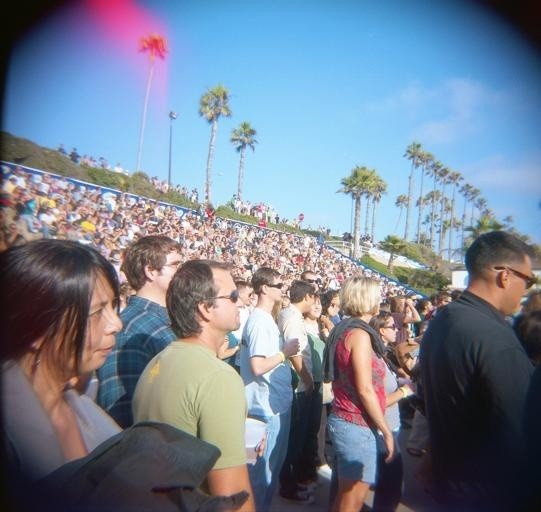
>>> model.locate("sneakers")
[279,478,319,505]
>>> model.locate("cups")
[247,418,269,466]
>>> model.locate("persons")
[0,129,541,510]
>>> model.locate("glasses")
[205,290,240,303]
[380,324,396,330]
[494,266,537,289]
[267,283,283,290]
[303,279,318,284]
